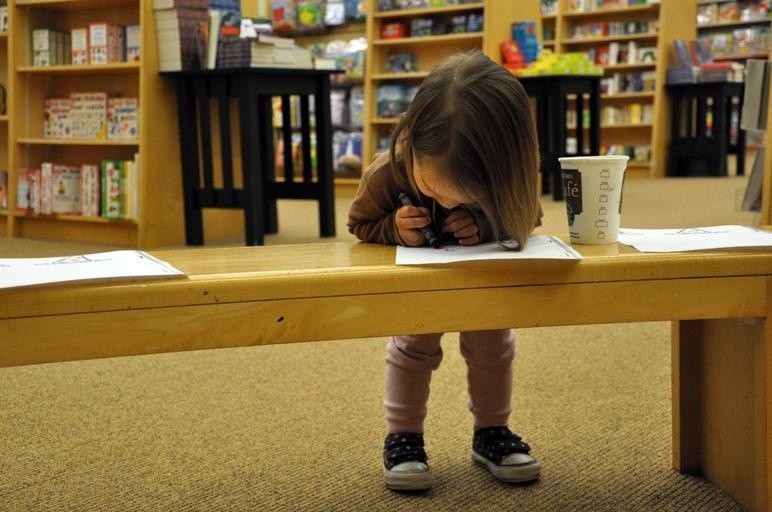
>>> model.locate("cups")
[558,155,630,245]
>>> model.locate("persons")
[346,47,544,493]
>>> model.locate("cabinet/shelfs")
[241,2,363,197]
[697,1,768,149]
[0,1,263,251]
[541,1,696,180]
[365,0,542,173]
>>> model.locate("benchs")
[0,225,772,511]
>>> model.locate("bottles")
[607,40,642,124]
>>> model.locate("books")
[374,2,482,157]
[155,0,336,72]
[665,2,771,145]
[499,1,660,162]
[2,0,141,222]
[268,1,368,178]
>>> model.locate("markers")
[395,188,440,249]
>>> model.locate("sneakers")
[382,430,434,492]
[471,425,543,485]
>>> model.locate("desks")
[668,82,746,177]
[157,68,346,246]
[517,75,614,201]
